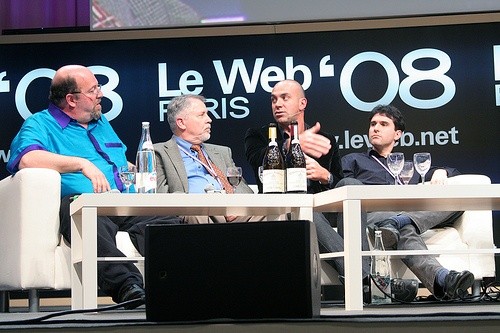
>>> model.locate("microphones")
[288,110,302,117]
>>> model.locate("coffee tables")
[314,183,500,311]
[70,191,314,316]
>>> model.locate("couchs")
[1,168,497,313]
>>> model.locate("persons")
[340,105,474,300]
[6,64,184,311]
[245,79,419,303]
[153,94,268,223]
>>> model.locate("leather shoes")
[367,219,399,248]
[391,278,419,302]
[445,270,474,299]
[121,284,147,308]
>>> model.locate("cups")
[258,166,264,185]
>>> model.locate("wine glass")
[413,152,432,186]
[398,161,415,185]
[387,152,404,186]
[118,164,136,194]
[226,166,242,194]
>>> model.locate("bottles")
[134,121,157,193]
[369,230,392,305]
[283,120,307,194]
[261,122,285,194]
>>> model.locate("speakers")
[145,219,323,321]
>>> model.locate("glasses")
[69,84,102,95]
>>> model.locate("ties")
[191,144,234,194]
